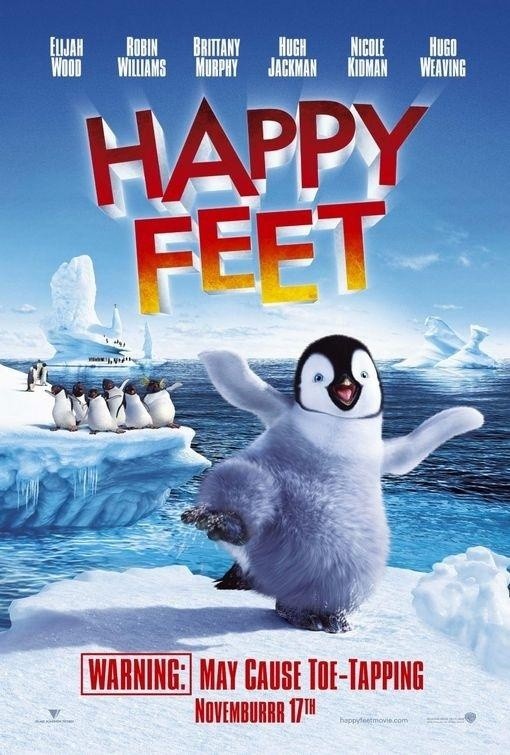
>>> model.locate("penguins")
[25,361,48,392]
[45,377,183,435]
[180,334,486,636]
